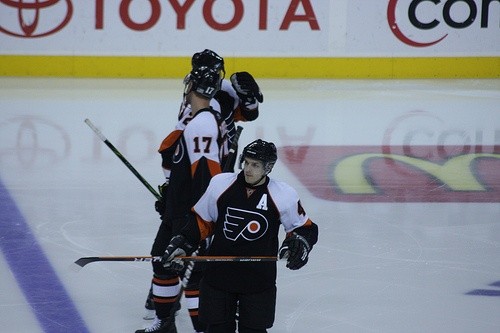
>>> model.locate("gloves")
[159,234,194,273]
[229,72,264,106]
[276,232,311,271]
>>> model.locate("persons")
[159,48,263,173]
[162,138,319,333]
[135,65,227,333]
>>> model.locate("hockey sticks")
[84,118,166,202]
[69,254,287,272]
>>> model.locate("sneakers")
[135,315,178,333]
[142,294,181,320]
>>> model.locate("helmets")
[187,66,220,101]
[239,140,277,174]
[191,48,224,71]
